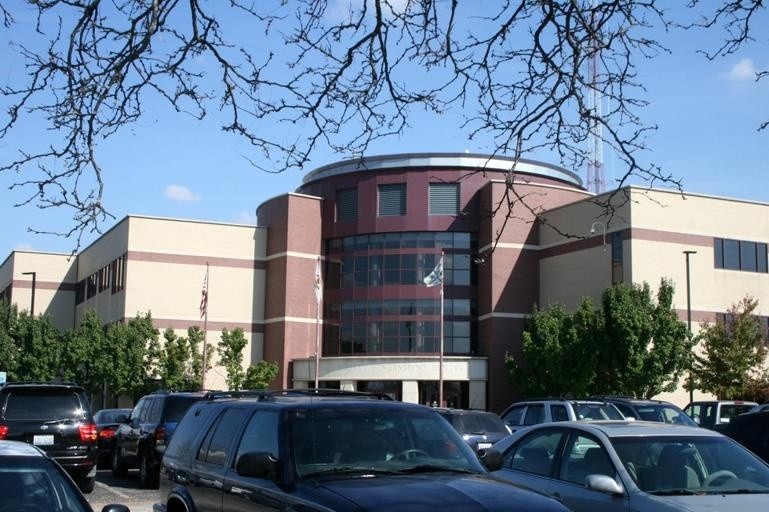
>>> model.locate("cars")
[431,408,515,464]
[481,420,769,512]
[93,408,133,467]
[712,405,769,462]
[0,438,131,512]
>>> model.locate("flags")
[198,271,209,320]
[423,257,443,288]
[313,259,323,303]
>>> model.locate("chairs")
[644,445,699,492]
[523,445,610,480]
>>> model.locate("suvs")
[1,382,100,493]
[586,396,702,428]
[496,397,632,457]
[112,390,235,488]
[154,388,575,512]
[673,400,761,431]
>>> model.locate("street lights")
[22,271,36,315]
[683,251,697,417]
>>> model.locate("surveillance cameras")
[590,229,595,234]
